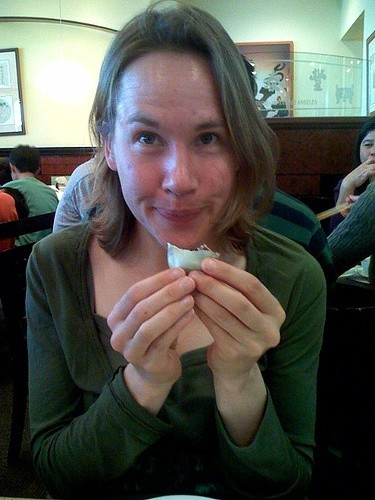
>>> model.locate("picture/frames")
[236,39,294,118]
[0,46,26,136]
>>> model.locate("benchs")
[0,213,56,477]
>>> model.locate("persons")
[327,182,375,293]
[329,116,375,238]
[253,177,332,280]
[1,187,28,252]
[52,156,105,235]
[26,0,327,500]
[3,146,59,247]
